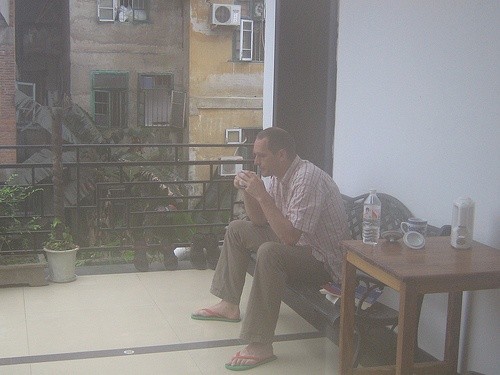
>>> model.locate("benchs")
[246,193,451,368]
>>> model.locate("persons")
[191,127,353,371]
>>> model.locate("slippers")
[225,351,276,371]
[191,308,240,321]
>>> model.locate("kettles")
[451,196,476,250]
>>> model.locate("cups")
[399,217,429,240]
[238,170,257,189]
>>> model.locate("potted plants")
[0,174,49,287]
[43,216,79,282]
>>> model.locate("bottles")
[361,189,383,245]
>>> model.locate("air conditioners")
[210,4,241,25]
[218,157,243,176]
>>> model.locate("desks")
[337,235,500,375]
